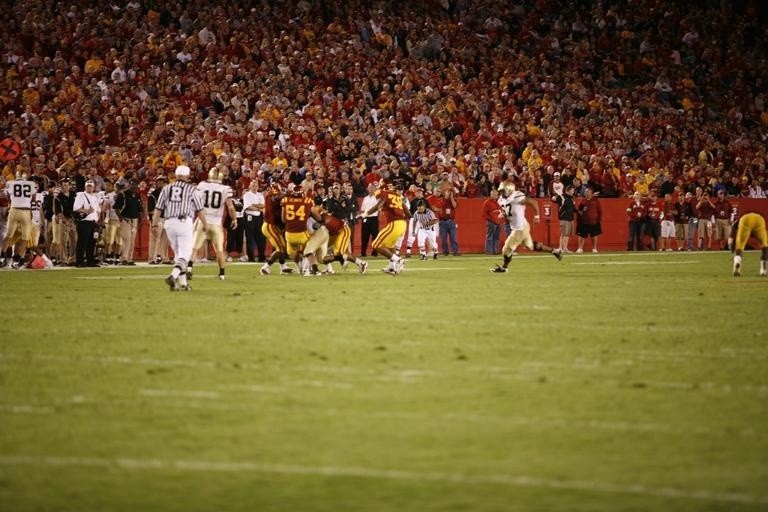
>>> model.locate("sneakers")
[489,266,508,272]
[553,249,561,261]
[164,272,192,291]
[259,260,367,277]
[382,256,407,276]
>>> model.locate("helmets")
[379,178,392,190]
[498,181,515,198]
[15,170,26,180]
[270,180,305,197]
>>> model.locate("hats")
[208,167,223,183]
[175,165,189,175]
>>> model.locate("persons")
[259,180,368,274]
[358,178,408,275]
[151,165,207,290]
[487,178,564,274]
[727,192,767,277]
[0,0,768,271]
[185,167,238,281]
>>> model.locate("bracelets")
[534,214,539,220]
[231,219,237,222]
[727,236,735,245]
[152,224,157,229]
